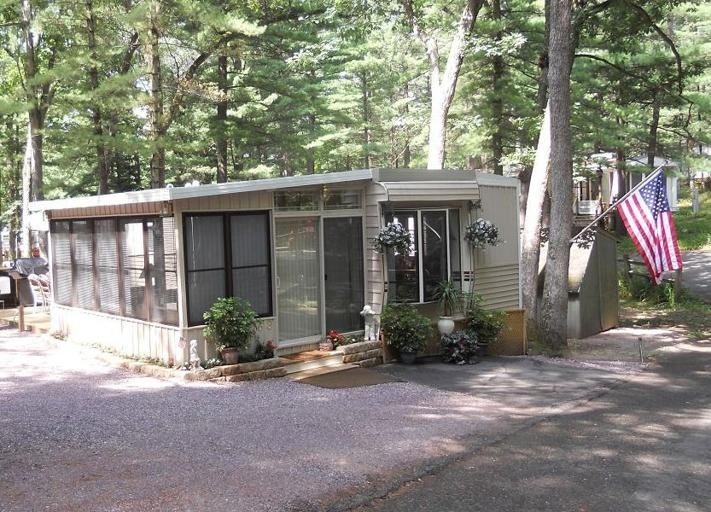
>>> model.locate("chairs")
[27,270,51,316]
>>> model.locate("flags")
[616,168,683,286]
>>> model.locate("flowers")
[374,217,419,257]
[464,217,506,250]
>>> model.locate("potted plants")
[380,278,513,366]
[201,295,266,365]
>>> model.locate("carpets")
[291,365,409,390]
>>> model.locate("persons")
[359,304,377,341]
